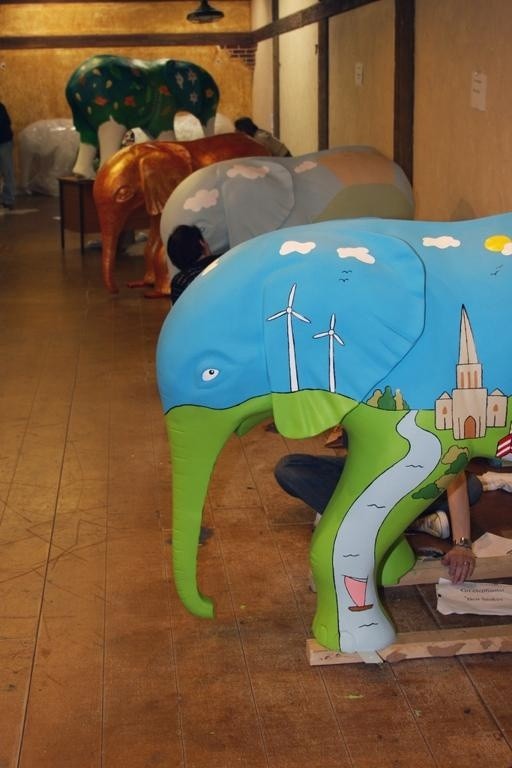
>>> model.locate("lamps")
[186,0,224,24]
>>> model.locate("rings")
[464,562,469,566]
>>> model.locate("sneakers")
[407,511,449,539]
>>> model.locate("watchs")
[452,537,472,549]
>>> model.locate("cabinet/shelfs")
[56,175,135,258]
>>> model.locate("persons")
[234,116,293,158]
[167,223,226,306]
[0,101,14,215]
[273,423,483,586]
